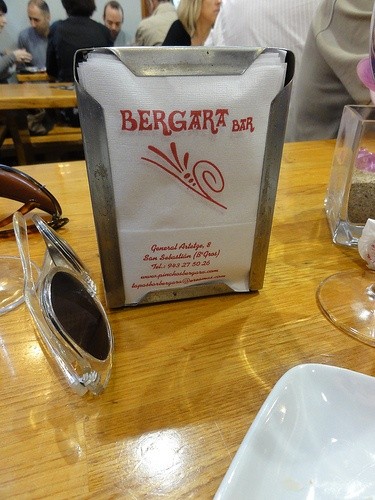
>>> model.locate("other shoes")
[26,108,48,135]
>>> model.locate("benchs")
[3,123,84,145]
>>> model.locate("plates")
[211,363,375,500]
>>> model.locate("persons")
[0,0,180,136]
[161,0,222,46]
[282,0,375,143]
[204,0,324,111]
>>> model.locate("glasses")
[0,164,69,238]
[0,211,114,398]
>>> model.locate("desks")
[0,132,375,500]
[0,69,78,109]
